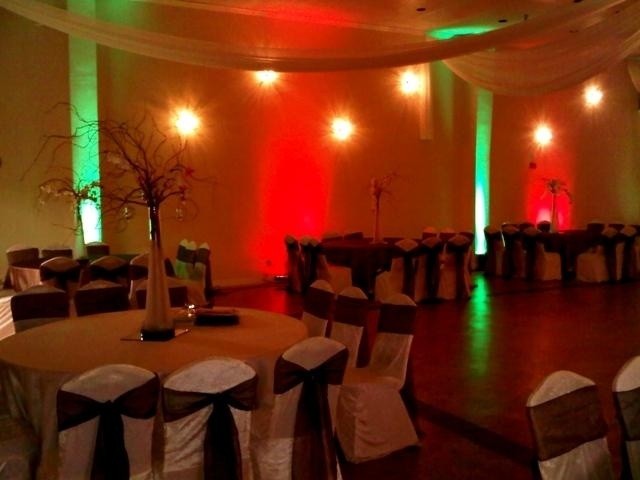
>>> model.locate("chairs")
[299,278,332,340]
[285,219,636,303]
[130,251,174,280]
[607,354,640,478]
[249,337,350,479]
[39,256,83,292]
[135,276,188,310]
[526,368,612,478]
[86,240,109,258]
[324,286,367,368]
[161,352,260,480]
[2,410,42,478]
[335,292,421,465]
[62,362,160,480]
[5,242,39,269]
[175,238,214,308]
[40,241,74,267]
[73,280,131,317]
[89,256,132,292]
[10,285,70,333]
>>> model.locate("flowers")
[19,88,212,238]
[28,167,104,219]
[366,166,407,205]
[538,175,571,198]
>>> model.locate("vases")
[372,198,382,243]
[547,195,561,233]
[70,205,88,262]
[137,202,176,340]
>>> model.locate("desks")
[1,306,307,478]
[7,254,139,290]
